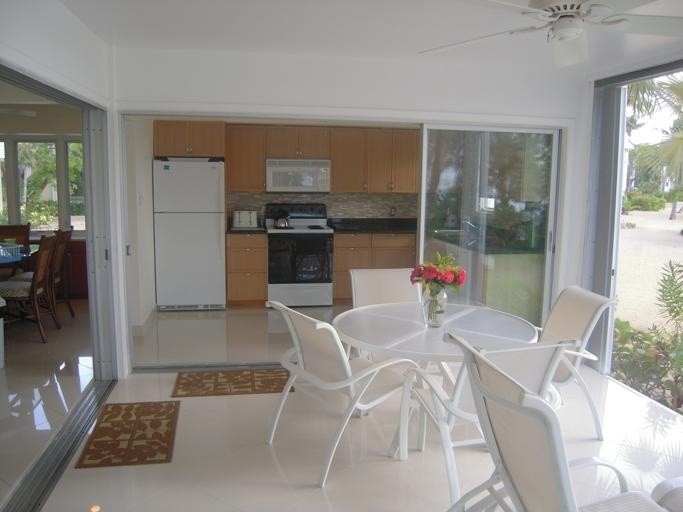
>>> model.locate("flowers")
[408,250,469,326]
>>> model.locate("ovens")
[267,233,334,307]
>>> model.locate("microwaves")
[265,158,330,194]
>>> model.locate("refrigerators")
[150,156,226,312]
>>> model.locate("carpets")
[75,400,181,469]
[170,367,295,397]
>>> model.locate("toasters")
[232,210,257,228]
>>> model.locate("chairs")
[350,267,433,419]
[444,286,615,450]
[443,330,683,512]
[266,302,421,488]
[0,223,76,350]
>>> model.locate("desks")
[332,303,538,456]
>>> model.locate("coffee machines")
[514,206,545,251]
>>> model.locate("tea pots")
[271,211,291,228]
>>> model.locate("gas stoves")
[266,219,334,234]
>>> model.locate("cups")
[0,239,22,255]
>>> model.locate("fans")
[414,1,683,62]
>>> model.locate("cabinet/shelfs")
[265,124,331,160]
[332,232,416,302]
[330,128,462,196]
[495,133,551,205]
[225,124,264,193]
[150,119,224,156]
[67,238,87,299]
[224,232,267,308]
